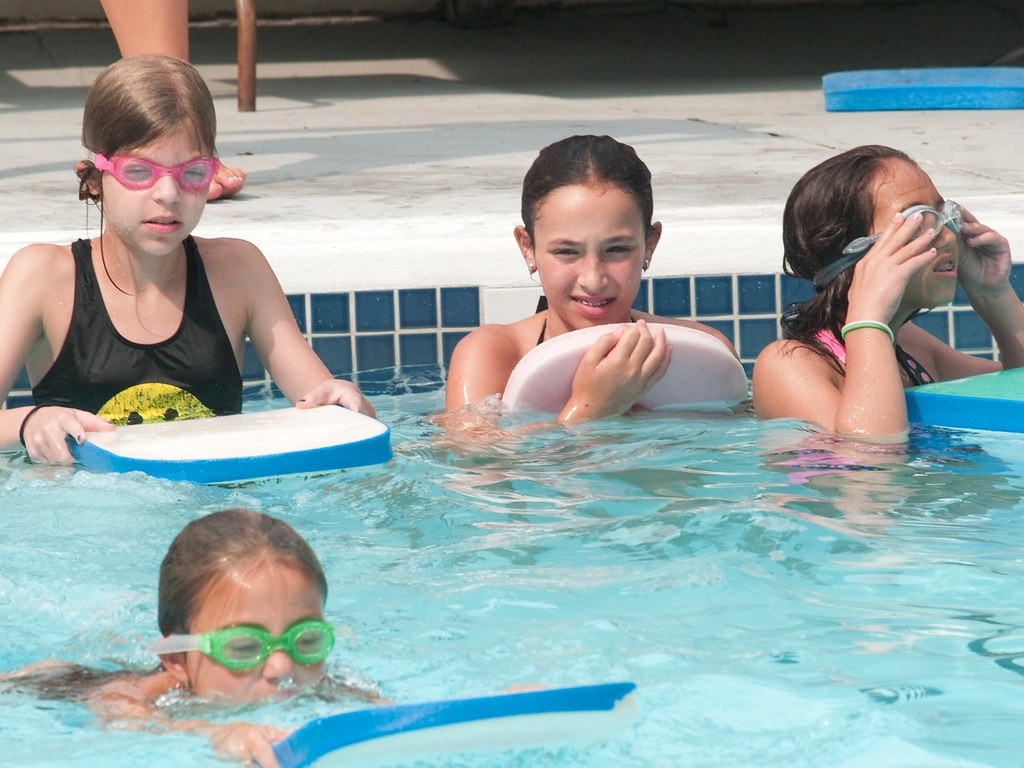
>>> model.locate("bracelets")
[840,320,895,344]
[20,404,53,448]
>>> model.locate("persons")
[1,509,548,768]
[445,134,751,443]
[103,0,250,202]
[751,144,1024,445]
[0,56,379,469]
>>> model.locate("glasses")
[81,145,220,194]
[154,617,336,673]
[812,200,963,293]
